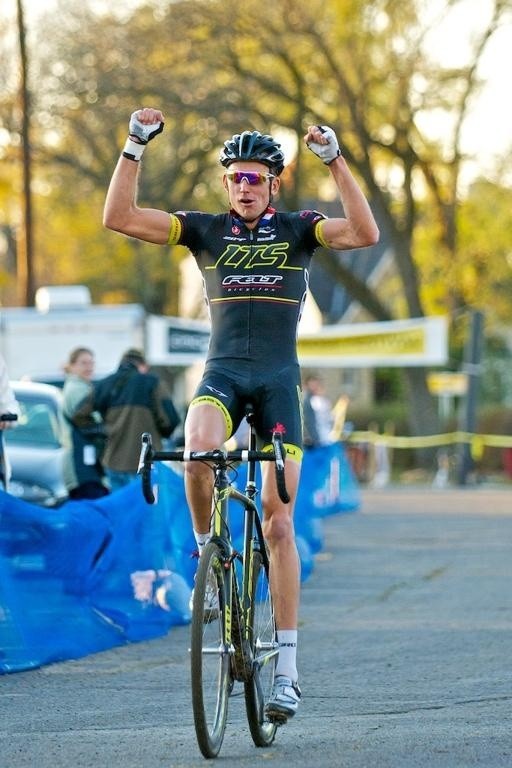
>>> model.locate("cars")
[2,376,80,578]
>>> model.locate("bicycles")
[136,403,290,757]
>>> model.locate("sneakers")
[263,673,302,717]
[188,563,220,620]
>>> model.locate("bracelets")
[122,135,148,163]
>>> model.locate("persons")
[102,107,381,720]
[310,375,340,447]
[71,347,181,494]
[58,346,110,498]
[299,367,322,447]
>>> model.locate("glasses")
[226,170,275,185]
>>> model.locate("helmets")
[218,130,285,174]
